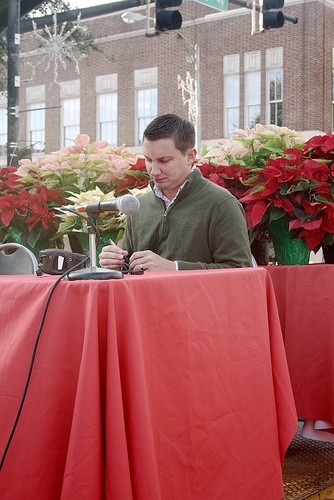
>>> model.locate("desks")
[0,261,298,500]
[260,263,334,441]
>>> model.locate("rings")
[140,264,143,270]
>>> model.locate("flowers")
[0,120,334,247]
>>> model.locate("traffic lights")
[155,0,183,33]
[258,0,285,32]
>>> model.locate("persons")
[98,114,253,272]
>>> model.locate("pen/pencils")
[109,238,129,262]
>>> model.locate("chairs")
[0,241,40,275]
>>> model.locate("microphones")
[85,194,140,214]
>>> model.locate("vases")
[269,220,309,266]
[8,226,49,258]
[67,232,116,267]
